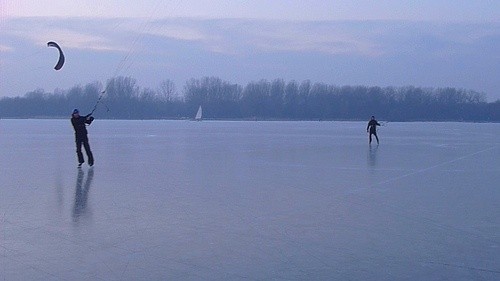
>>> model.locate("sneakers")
[79,161,84,166]
[89,163,93,166]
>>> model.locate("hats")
[72,108,79,114]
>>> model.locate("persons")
[71,108,95,166]
[366,114,381,144]
